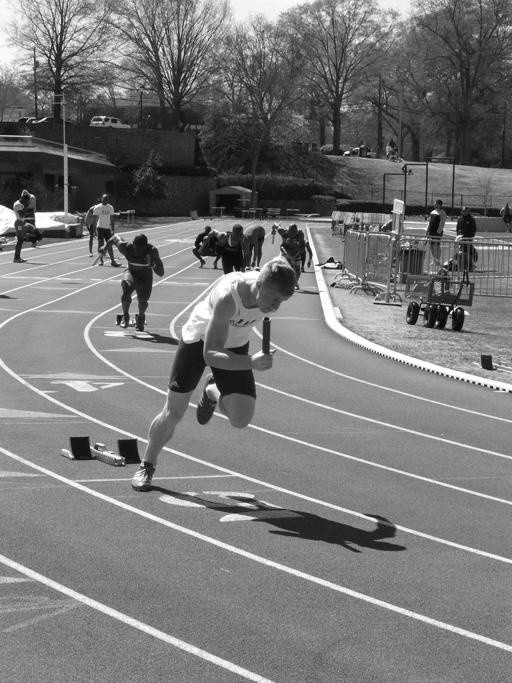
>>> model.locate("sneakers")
[131,461,155,491]
[89,253,122,266]
[13,258,28,262]
[196,373,221,425]
[120,315,144,332]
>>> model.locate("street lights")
[31,41,39,120]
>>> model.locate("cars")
[31,116,73,126]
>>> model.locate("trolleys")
[406,275,475,329]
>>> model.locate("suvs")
[90,114,131,128]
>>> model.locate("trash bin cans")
[399,249,426,284]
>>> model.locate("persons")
[99,232,164,330]
[299,239,313,273]
[271,221,305,291]
[132,257,296,491]
[499,203,511,232]
[93,194,124,266]
[12,190,43,262]
[85,197,106,256]
[426,199,446,264]
[193,224,266,277]
[456,205,478,270]
